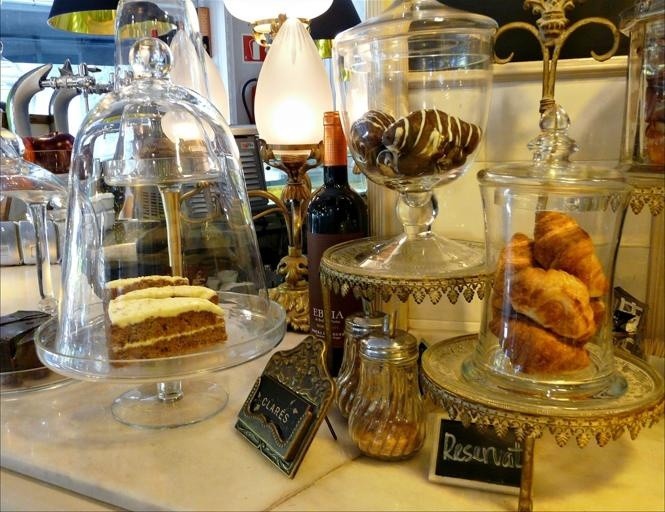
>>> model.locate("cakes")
[108,285,228,361]
[112,285,219,305]
[102,276,190,313]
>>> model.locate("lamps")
[254,19,333,207]
[223,0,334,48]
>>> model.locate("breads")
[489,213,608,375]
[353,108,482,175]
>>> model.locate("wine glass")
[332,29,493,278]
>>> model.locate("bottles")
[307,110,371,378]
[462,105,631,401]
[349,311,427,462]
[334,300,388,419]
